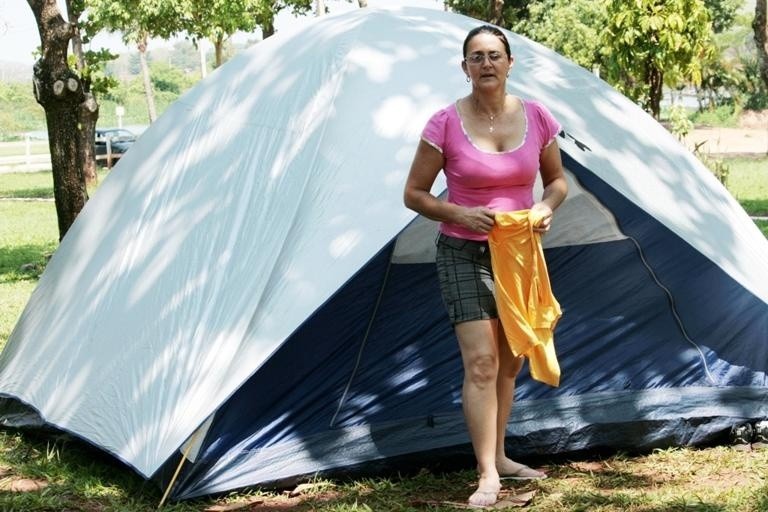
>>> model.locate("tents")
[1,3,768,505]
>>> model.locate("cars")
[94,129,136,167]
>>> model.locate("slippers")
[466,482,505,510]
[498,466,548,482]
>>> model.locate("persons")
[403,24,572,509]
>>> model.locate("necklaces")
[484,113,503,135]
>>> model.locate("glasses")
[462,52,511,64]
